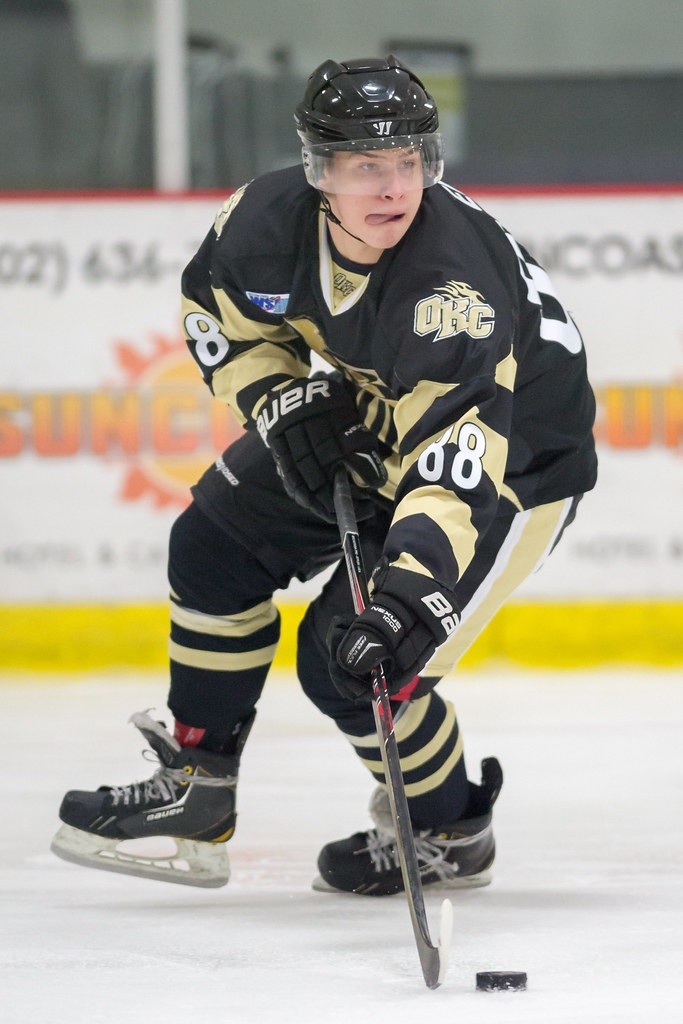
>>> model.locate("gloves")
[256,377,389,524]
[325,556,461,707]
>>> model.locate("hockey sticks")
[333,464,456,990]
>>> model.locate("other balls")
[476,970,528,991]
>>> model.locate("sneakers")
[50,708,257,889]
[311,756,503,897]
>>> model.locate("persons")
[50,53,599,898]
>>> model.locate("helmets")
[293,55,441,180]
